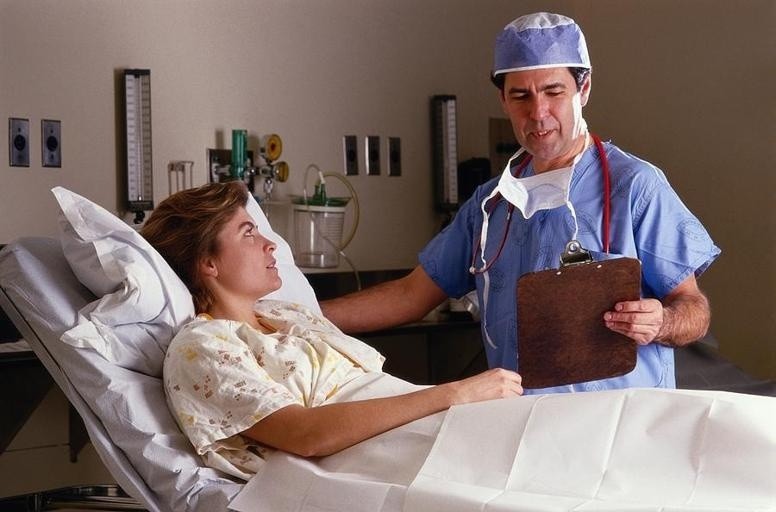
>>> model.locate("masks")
[498,129,590,220]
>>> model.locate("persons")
[139,182,524,483]
[318,13,722,395]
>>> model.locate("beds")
[1,182,430,511]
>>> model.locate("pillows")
[43,182,340,382]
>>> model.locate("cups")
[292,208,345,269]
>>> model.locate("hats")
[492,12,592,78]
